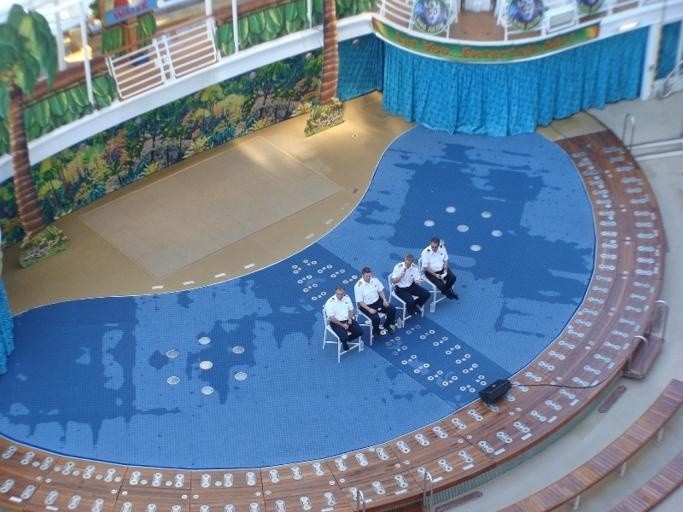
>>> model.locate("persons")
[325,285,363,351]
[391,255,430,315]
[354,267,396,340]
[421,238,459,300]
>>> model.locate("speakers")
[478,379,511,404]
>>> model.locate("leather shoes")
[414,304,421,314]
[406,305,412,315]
[342,341,349,351]
[441,288,458,300]
[384,323,393,335]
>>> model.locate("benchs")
[497,376,682,512]
[603,446,682,512]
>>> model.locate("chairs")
[385,272,428,329]
[318,308,363,366]
[353,295,393,346]
[415,261,456,313]
